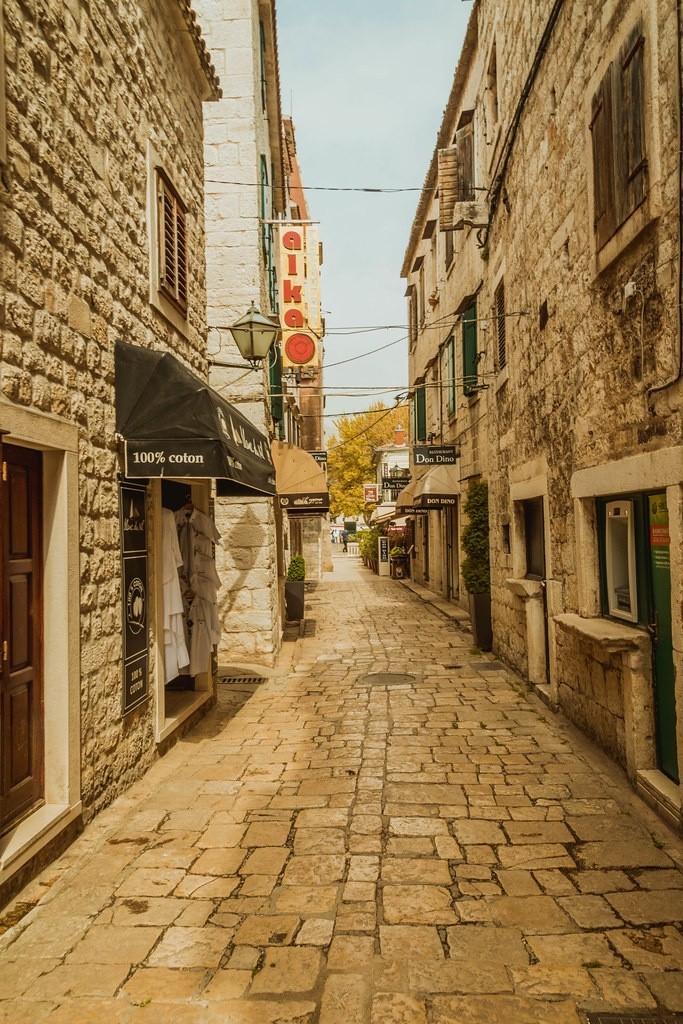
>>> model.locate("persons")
[331,529,342,544]
[341,530,348,553]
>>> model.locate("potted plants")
[359,527,382,574]
[285,555,306,621]
[461,479,494,652]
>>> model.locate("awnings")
[395,480,428,515]
[413,458,459,510]
[369,507,410,524]
[115,339,276,497]
[270,439,329,513]
